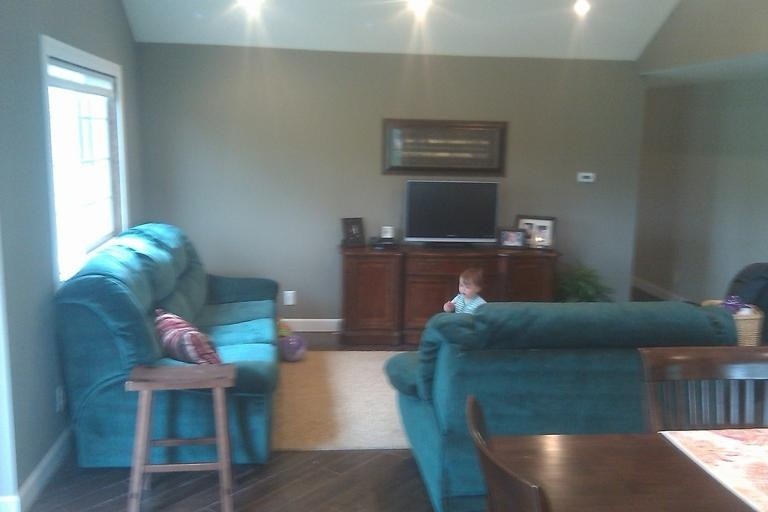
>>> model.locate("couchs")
[54,219,279,474]
[382,294,740,511]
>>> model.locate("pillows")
[151,304,222,367]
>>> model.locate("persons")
[443,267,487,314]
[504,232,521,246]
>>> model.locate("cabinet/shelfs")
[336,243,560,348]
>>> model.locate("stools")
[123,363,237,512]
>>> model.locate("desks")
[489,427,768,511]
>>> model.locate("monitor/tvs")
[403,178,501,248]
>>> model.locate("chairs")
[460,393,544,512]
[636,343,767,431]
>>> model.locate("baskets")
[703,299,761,347]
